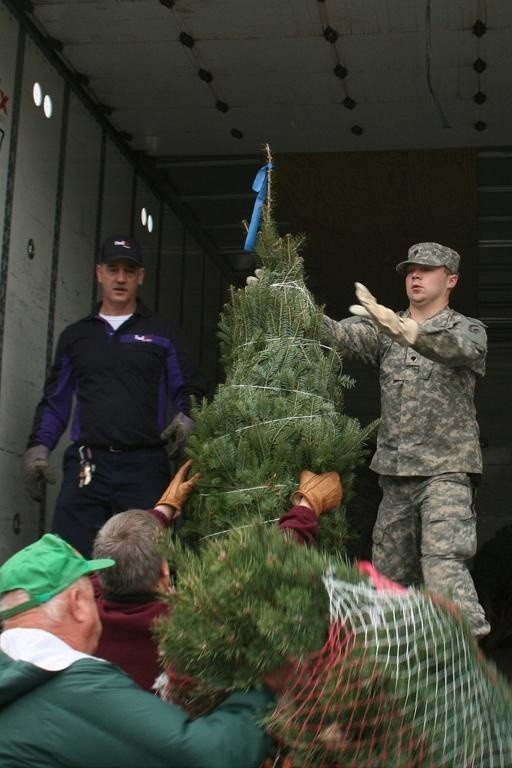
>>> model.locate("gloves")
[347,280,420,348]
[153,455,202,521]
[21,445,58,504]
[292,465,345,520]
[246,266,267,287]
[159,410,198,460]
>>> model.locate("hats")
[396,240,461,275]
[0,531,117,622]
[98,234,140,266]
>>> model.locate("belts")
[75,439,137,454]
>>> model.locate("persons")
[85,452,347,700]
[0,532,286,768]
[246,234,491,668]
[22,233,194,563]
